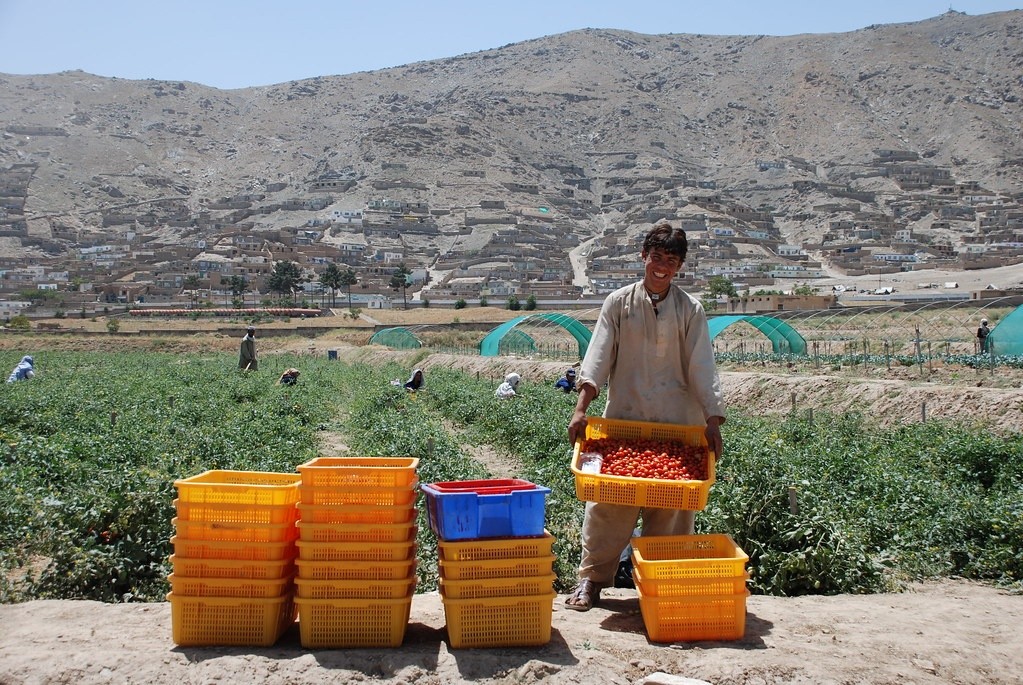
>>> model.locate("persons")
[977,318,991,355]
[280,368,300,385]
[495,373,520,398]
[555,368,576,393]
[8,355,36,384]
[563,223,727,613]
[403,369,426,393]
[238,326,258,371]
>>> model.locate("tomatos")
[585,434,708,509]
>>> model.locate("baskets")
[569,416,716,511]
[166,456,556,649]
[629,533,750,642]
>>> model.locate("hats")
[248,326,255,331]
[566,368,576,377]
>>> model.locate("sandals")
[562,580,602,611]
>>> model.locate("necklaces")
[644,285,670,300]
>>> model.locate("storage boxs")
[628,532,753,643]
[568,415,716,510]
[168,456,561,650]
